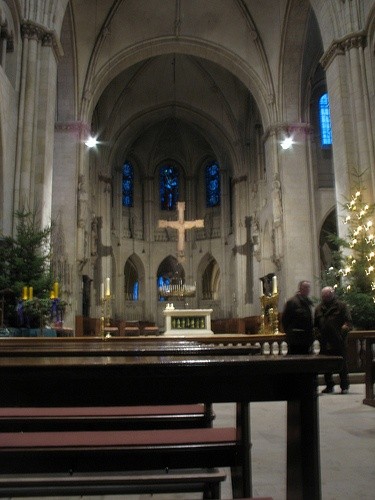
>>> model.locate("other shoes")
[322,386,333,392]
[340,387,349,394]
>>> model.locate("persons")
[284,280,316,355]
[313,287,353,394]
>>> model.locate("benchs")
[1,327,374,500]
